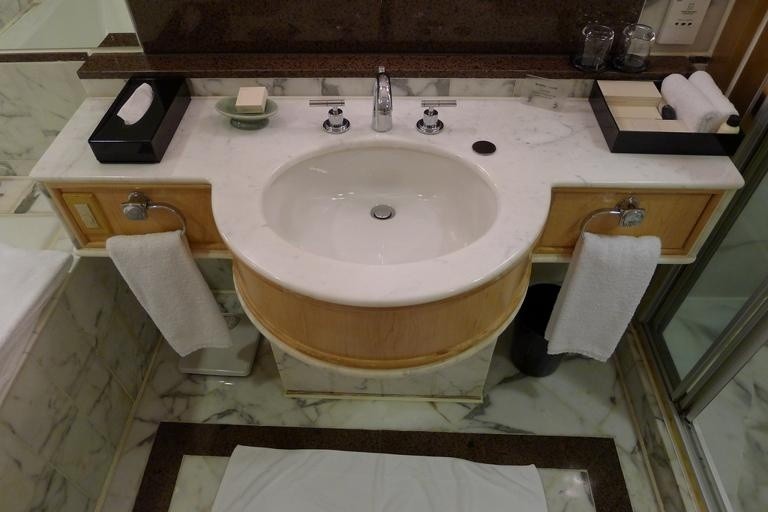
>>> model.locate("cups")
[573,25,614,70]
[617,23,656,71]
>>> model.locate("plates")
[214,98,279,131]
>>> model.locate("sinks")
[260,137,501,268]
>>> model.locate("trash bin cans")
[513,283,562,377]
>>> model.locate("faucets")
[371,65,394,133]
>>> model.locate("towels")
[661,73,721,133]
[104,229,233,357]
[689,70,740,123]
[545,232,662,365]
